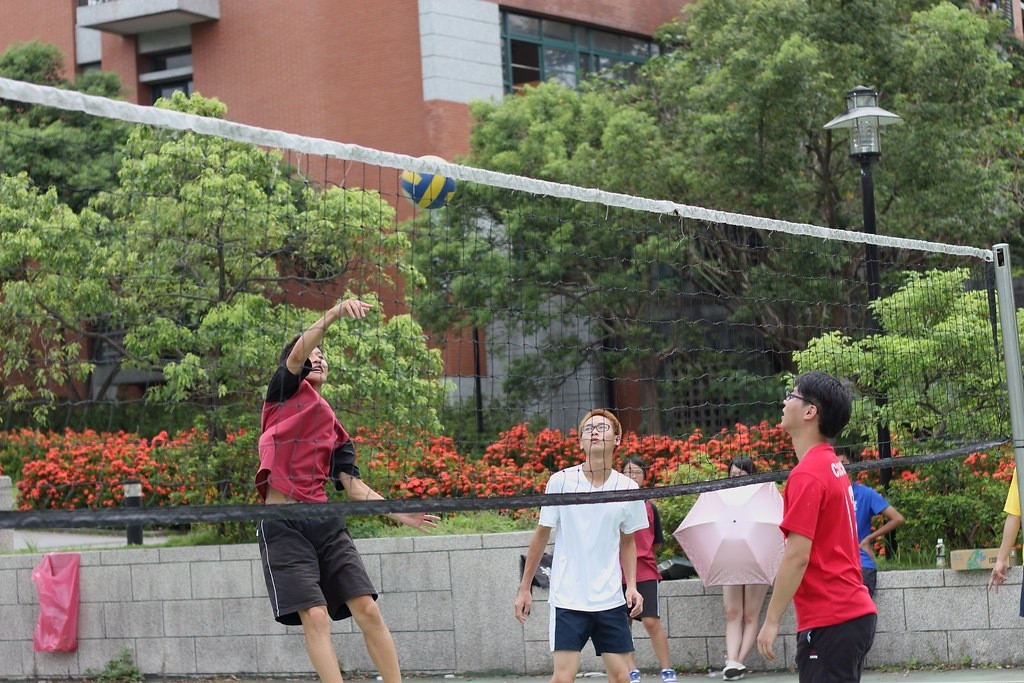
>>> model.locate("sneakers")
[722,655,747,678]
[629,670,641,683]
[723,673,744,681]
[661,668,678,683]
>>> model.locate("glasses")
[581,424,611,433]
[786,390,820,414]
[623,470,644,476]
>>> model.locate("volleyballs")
[399,154,457,210]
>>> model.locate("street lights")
[824,86,902,563]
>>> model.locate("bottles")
[1010,543,1017,565]
[936,538,946,568]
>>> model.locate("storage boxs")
[950,547,1017,570]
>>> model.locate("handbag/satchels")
[656,556,693,580]
[32,552,80,653]
[521,553,553,587]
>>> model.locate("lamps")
[121,479,143,497]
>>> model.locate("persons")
[723,457,769,680]
[835,446,903,596]
[620,457,676,683]
[256,298,441,683]
[757,373,878,683]
[989,467,1024,617]
[515,410,649,683]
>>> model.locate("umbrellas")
[672,479,786,587]
